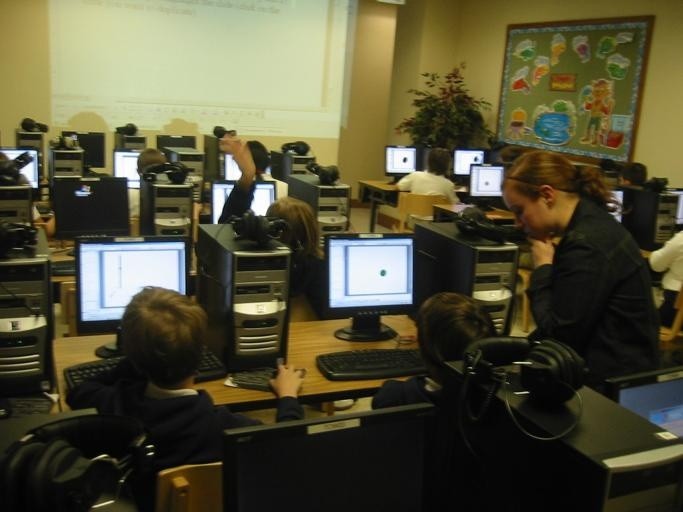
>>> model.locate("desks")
[52,315,419,417]
[433,204,515,224]
[358,180,468,234]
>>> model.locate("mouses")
[270,359,284,380]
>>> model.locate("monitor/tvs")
[384,145,425,176]
[606,190,623,223]
[156,134,199,151]
[48,175,131,256]
[664,187,683,232]
[224,153,272,181]
[468,163,513,201]
[112,148,154,189]
[0,147,40,192]
[323,231,421,343]
[60,130,105,176]
[73,236,192,337]
[211,180,277,225]
[452,149,485,177]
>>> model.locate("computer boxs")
[16,132,45,180]
[197,223,293,370]
[204,135,224,183]
[440,352,683,510]
[288,173,353,249]
[0,256,54,403]
[114,135,148,150]
[0,200,39,258]
[48,149,86,179]
[410,218,521,339]
[271,150,320,182]
[616,187,680,254]
[140,182,194,252]
[165,147,206,203]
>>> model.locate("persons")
[43,172,108,237]
[396,148,460,204]
[503,150,658,401]
[138,149,167,178]
[499,145,522,167]
[1,152,45,227]
[65,286,313,497]
[616,160,646,190]
[218,134,323,283]
[245,141,289,200]
[648,226,683,327]
[372,292,498,408]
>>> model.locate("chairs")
[513,269,535,332]
[659,285,683,342]
[393,192,450,233]
[55,281,79,337]
[158,462,223,512]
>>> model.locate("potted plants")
[395,61,494,175]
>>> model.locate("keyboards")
[53,256,78,279]
[61,344,229,399]
[314,349,434,381]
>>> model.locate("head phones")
[21,118,49,132]
[454,206,519,243]
[461,335,585,403]
[3,412,132,511]
[459,335,585,442]
[282,140,312,155]
[644,176,669,192]
[213,125,239,138]
[230,214,288,252]
[141,162,189,185]
[304,163,340,185]
[124,122,138,129]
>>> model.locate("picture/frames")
[496,14,655,169]
